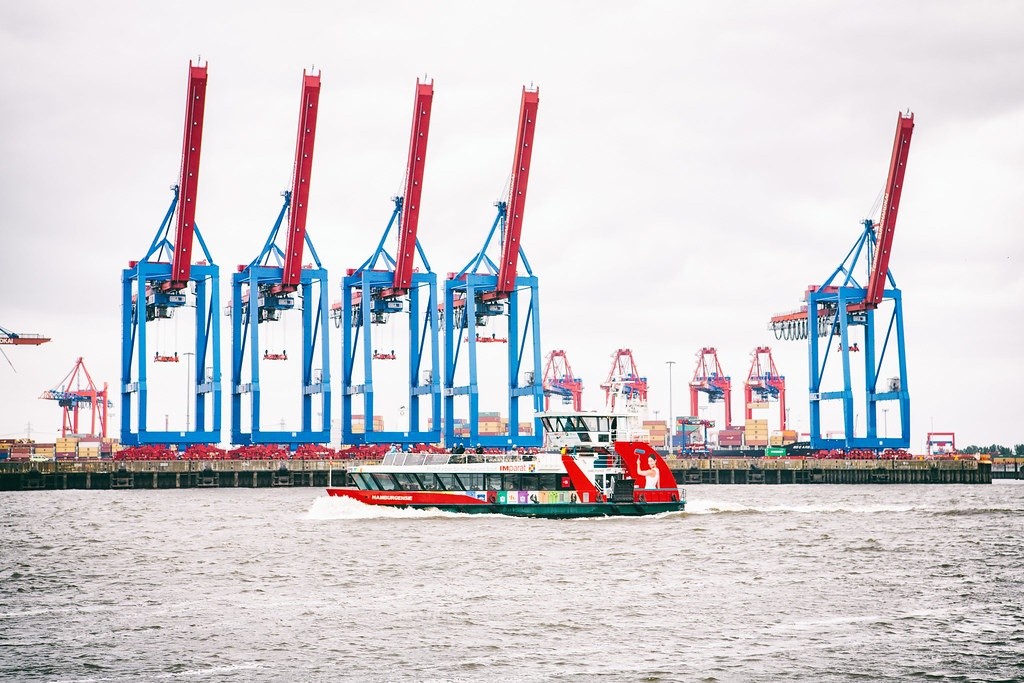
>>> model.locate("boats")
[326,406,687,520]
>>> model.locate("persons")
[637,454,660,489]
[390,441,571,461]
